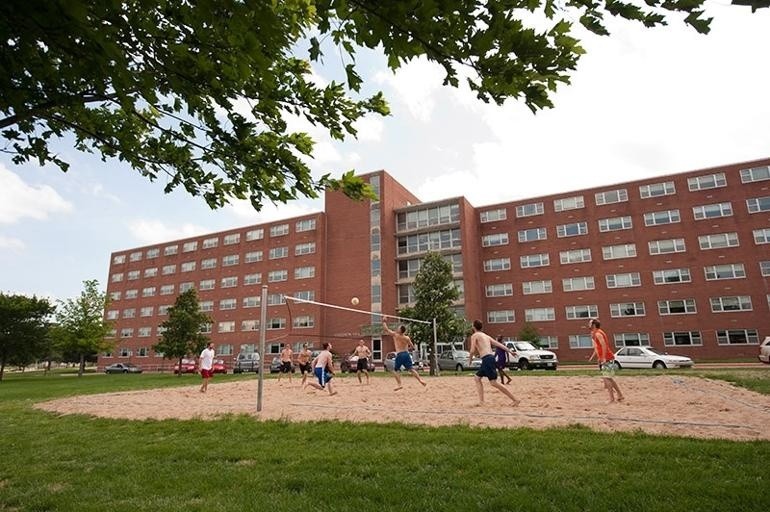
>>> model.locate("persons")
[586,318,625,405]
[274,343,294,385]
[304,342,338,396]
[348,338,373,386]
[380,315,427,391]
[198,341,216,393]
[492,334,513,384]
[296,343,312,388]
[468,320,521,408]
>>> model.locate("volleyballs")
[351,297,359,305]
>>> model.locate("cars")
[436,350,484,373]
[341,354,377,374]
[614,346,694,370]
[173,357,198,375]
[309,350,334,369]
[213,357,228,375]
[102,363,144,374]
[269,355,296,374]
[383,351,425,373]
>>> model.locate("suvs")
[232,352,260,374]
[493,340,559,371]
[757,334,770,363]
